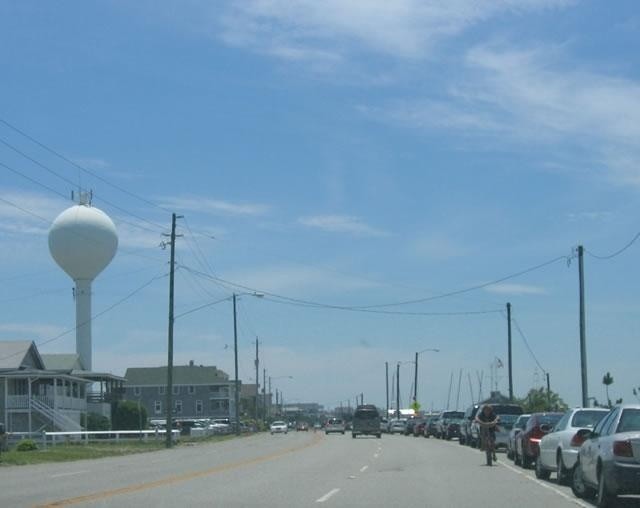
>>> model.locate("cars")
[572,405,640,507]
[515,411,565,468]
[150,417,325,436]
[380,410,465,440]
[508,414,532,460]
[325,418,345,434]
[535,408,610,485]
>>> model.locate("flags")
[496,357,504,369]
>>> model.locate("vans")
[352,405,381,439]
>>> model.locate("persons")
[474,403,499,462]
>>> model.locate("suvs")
[459,405,480,445]
[470,403,524,450]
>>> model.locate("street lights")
[414,349,440,401]
[268,375,294,417]
[232,291,264,435]
